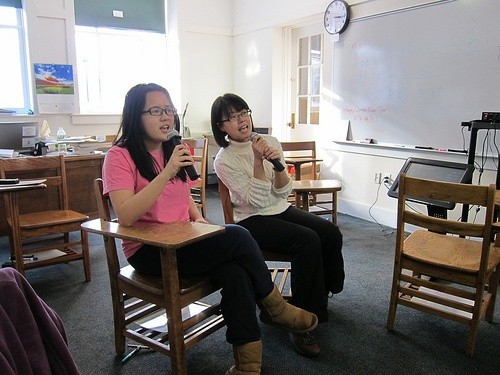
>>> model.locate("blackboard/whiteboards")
[333,1,500,158]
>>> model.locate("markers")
[361,141,377,144]
[448,149,468,152]
[415,146,435,151]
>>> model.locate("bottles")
[57,127,67,153]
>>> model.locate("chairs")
[386,172,500,356]
[0,139,341,375]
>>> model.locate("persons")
[101,83,319,375]
[211,93,345,361]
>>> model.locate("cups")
[48,145,55,151]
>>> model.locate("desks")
[0,150,112,236]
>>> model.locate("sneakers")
[289,328,320,356]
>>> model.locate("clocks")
[324,0,350,35]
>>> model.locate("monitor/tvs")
[387,157,474,210]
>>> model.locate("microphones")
[249,132,285,172]
[167,129,198,181]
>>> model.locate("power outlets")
[383,174,391,185]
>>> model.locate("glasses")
[217,108,252,122]
[142,106,177,116]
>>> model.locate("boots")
[225,338,263,375]
[258,283,318,333]
